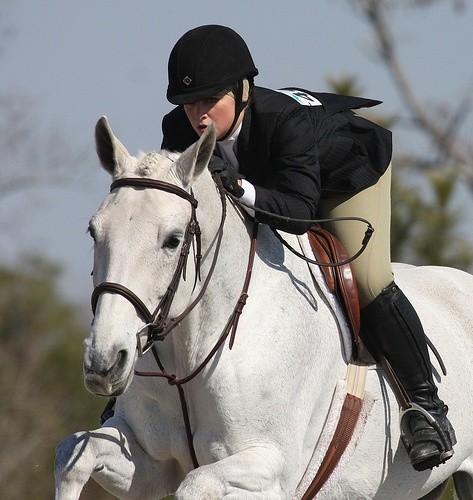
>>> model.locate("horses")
[52,115,472,499]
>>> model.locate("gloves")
[208,154,245,198]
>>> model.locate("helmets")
[164,24,259,106]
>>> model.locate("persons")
[162,22,460,472]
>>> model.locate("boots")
[361,282,457,469]
